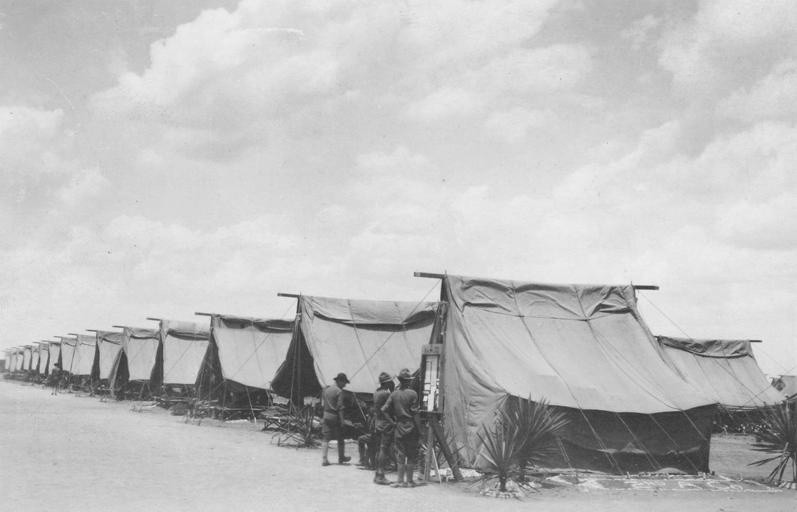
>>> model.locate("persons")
[372,371,394,484]
[354,407,381,467]
[379,367,427,488]
[322,374,352,466]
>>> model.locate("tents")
[653,333,790,432]
[771,373,796,402]
[2,311,293,421]
[271,293,439,443]
[414,269,718,472]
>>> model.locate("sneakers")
[339,456,351,462]
[374,474,416,486]
[321,461,328,465]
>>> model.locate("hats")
[397,368,415,380]
[333,374,349,382]
[380,371,394,383]
